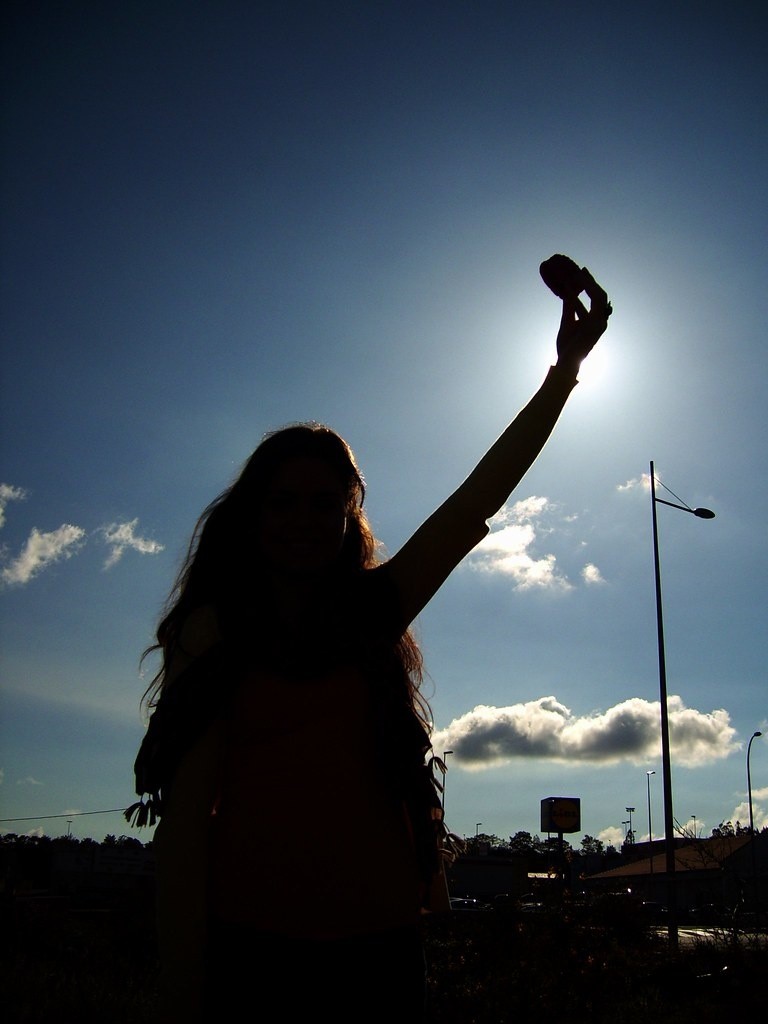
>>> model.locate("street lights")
[747,731,762,830]
[440,750,454,812]
[646,770,656,871]
[649,458,715,952]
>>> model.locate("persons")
[135,266,611,1024]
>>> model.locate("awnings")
[585,832,754,879]
